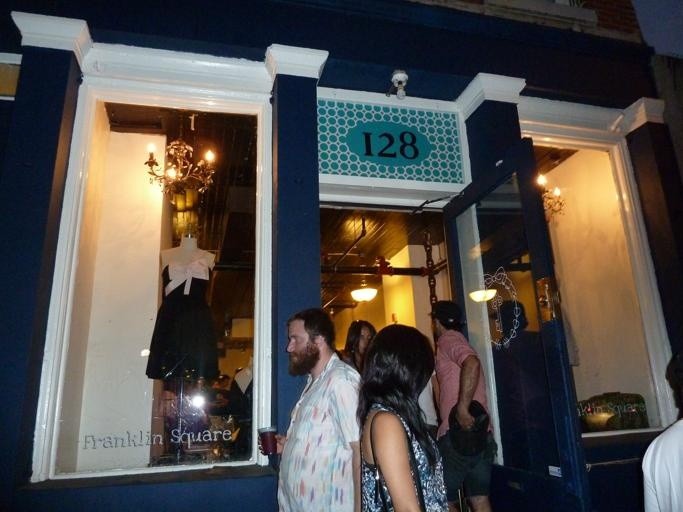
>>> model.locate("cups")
[258,427,277,456]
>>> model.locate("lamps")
[386,69,409,100]
[350,253,378,302]
[145,112,217,205]
[537,174,565,223]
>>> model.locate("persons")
[159,353,244,465]
[226,354,253,461]
[255,300,497,512]
[489,300,557,473]
[641,352,683,512]
[146,232,219,386]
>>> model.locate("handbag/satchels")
[450,399,490,454]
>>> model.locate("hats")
[429,300,460,323]
